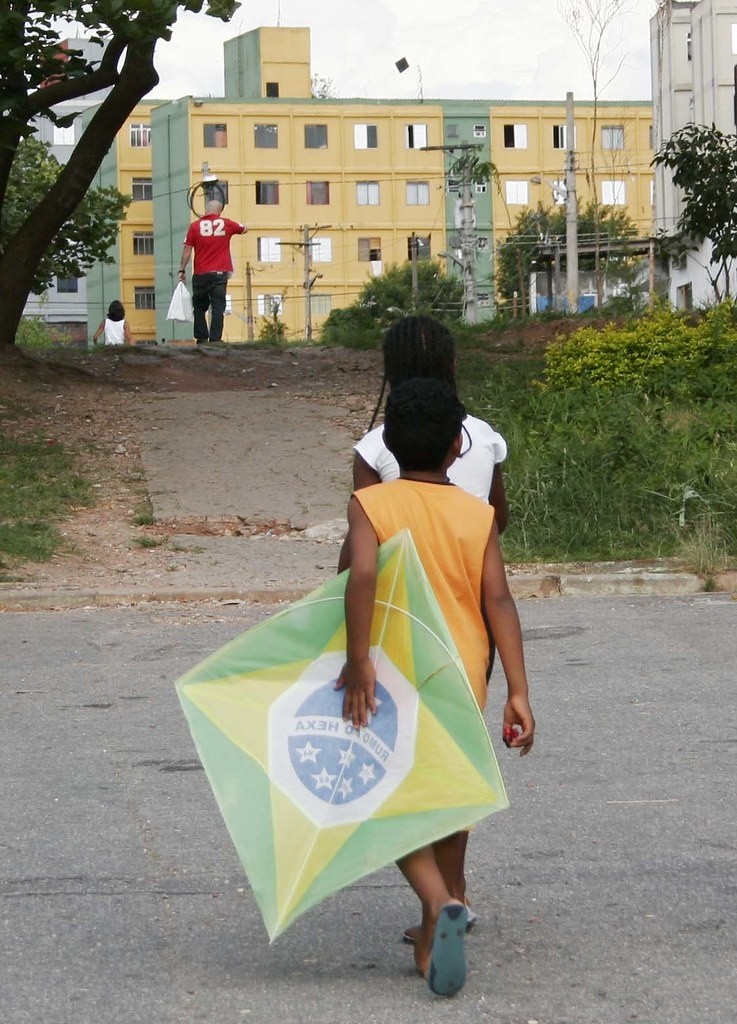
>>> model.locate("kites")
[170,524,514,951]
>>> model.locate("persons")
[179,199,249,344]
[92,300,135,346]
[337,319,513,949]
[337,382,537,999]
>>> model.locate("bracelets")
[179,270,184,272]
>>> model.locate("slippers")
[426,903,468,997]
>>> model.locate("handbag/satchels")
[165,281,193,322]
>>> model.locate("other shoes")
[403,923,419,945]
[197,339,224,344]
